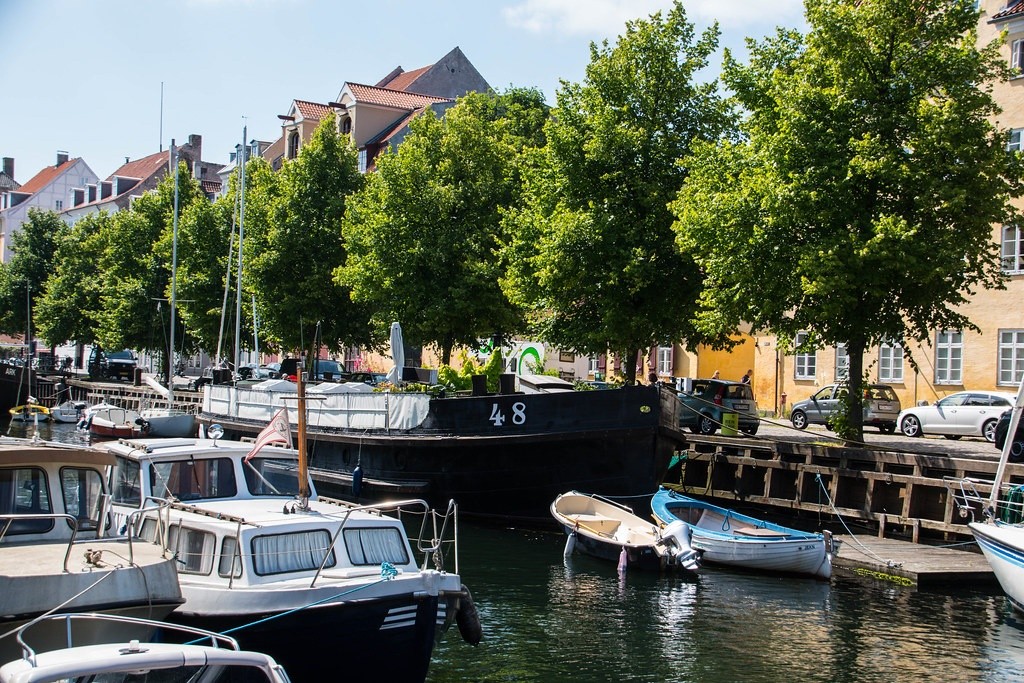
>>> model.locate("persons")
[865,391,872,398]
[836,390,847,398]
[741,370,753,397]
[218,356,229,368]
[693,387,703,394]
[712,370,719,379]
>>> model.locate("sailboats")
[8,279,50,421]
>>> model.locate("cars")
[897,390,1024,443]
[348,371,390,387]
[994,406,1024,463]
[251,368,281,378]
[589,381,615,390]
[238,367,254,380]
[674,379,760,434]
[265,363,281,371]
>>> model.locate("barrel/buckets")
[38,352,52,370]
[211,368,231,385]
[719,412,739,436]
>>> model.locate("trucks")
[279,358,350,385]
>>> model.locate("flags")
[245,406,292,461]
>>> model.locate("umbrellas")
[386,322,403,387]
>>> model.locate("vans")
[88,347,136,381]
[790,384,901,434]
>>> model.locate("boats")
[51,400,89,422]
[551,490,705,576]
[0,435,185,641]
[83,400,139,437]
[651,485,842,579]
[193,371,623,510]
[140,152,196,436]
[0,612,291,683]
[966,520,1024,605]
[84,436,461,653]
